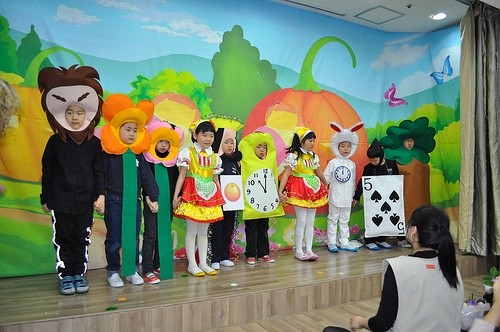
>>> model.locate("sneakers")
[125,272,144,285]
[107,272,124,288]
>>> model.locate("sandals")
[74,274,89,292]
[59,276,75,295]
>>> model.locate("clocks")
[245,167,281,212]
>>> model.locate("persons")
[466,277,500,332]
[323,138,360,253]
[236,136,290,264]
[141,129,183,285]
[394,135,426,248]
[210,136,242,270]
[321,205,462,332]
[39,97,106,295]
[93,112,160,287]
[278,127,328,260]
[352,145,399,250]
[178,122,225,276]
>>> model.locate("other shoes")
[396,238,412,248]
[199,263,218,275]
[258,254,275,263]
[153,267,160,274]
[211,262,221,269]
[295,252,309,260]
[246,257,256,264]
[187,265,205,277]
[340,243,359,251]
[364,242,381,250]
[327,243,339,252]
[220,260,235,266]
[379,242,393,248]
[305,251,319,260]
[144,272,160,284]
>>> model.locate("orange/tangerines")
[224,182,240,202]
